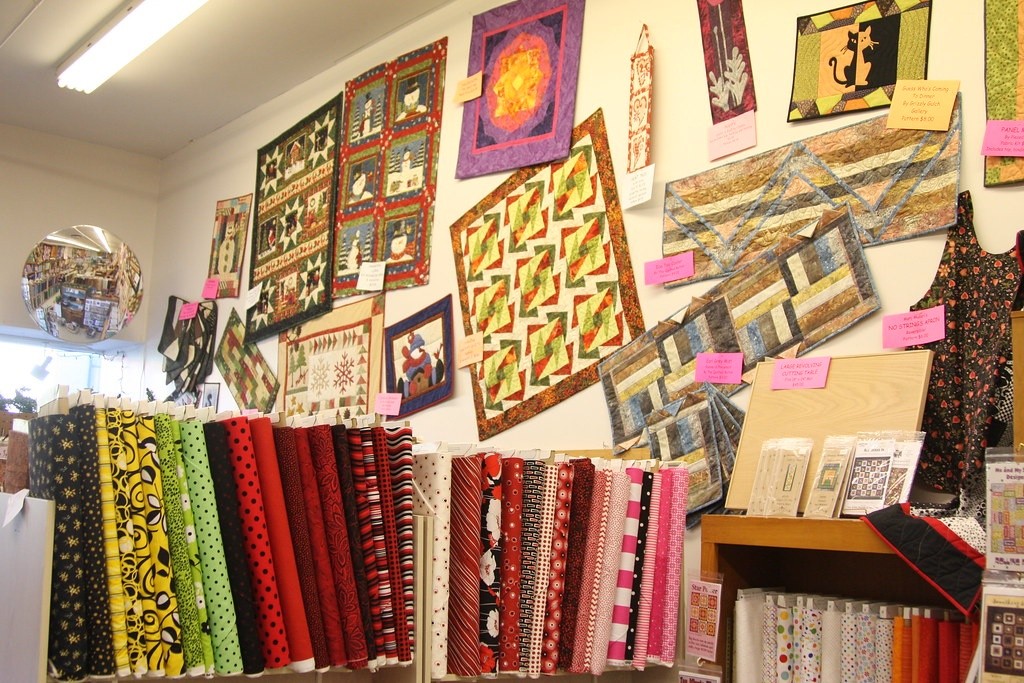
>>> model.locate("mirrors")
[20,225,144,343]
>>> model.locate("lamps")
[55,0,206,95]
